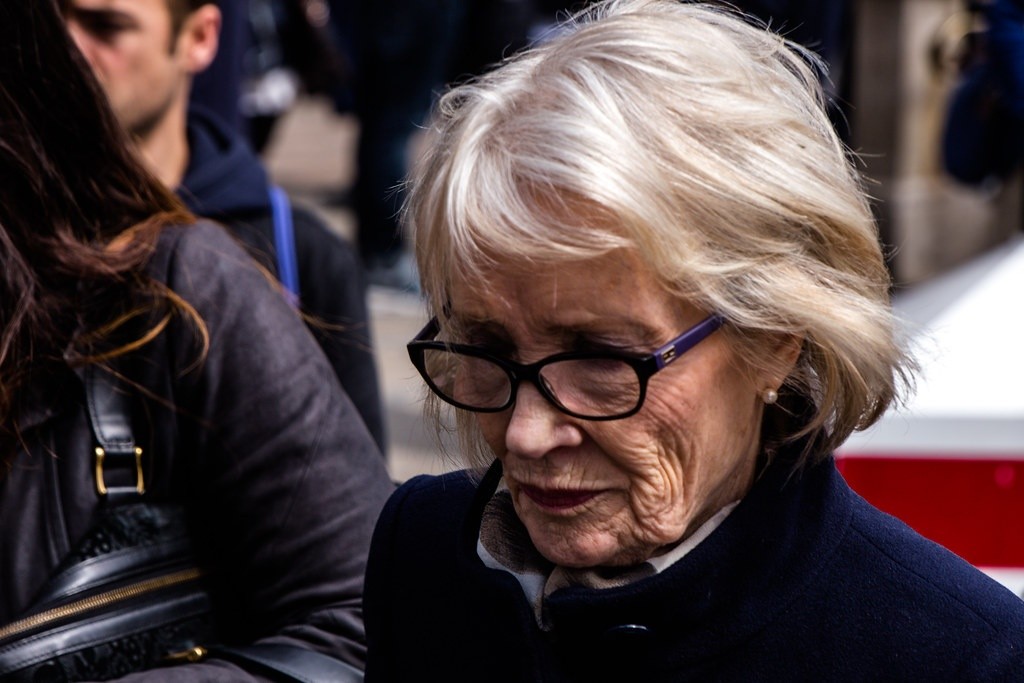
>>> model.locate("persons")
[0,0,398,683]
[363,0,1024,683]
[56,0,387,462]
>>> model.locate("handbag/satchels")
[0,220,222,683]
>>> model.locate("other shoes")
[359,250,426,290]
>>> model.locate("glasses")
[406,303,728,421]
